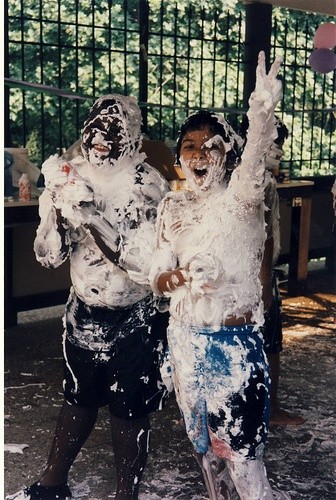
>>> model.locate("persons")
[148,51,293,500]
[214,98,309,424]
[5,91,184,500]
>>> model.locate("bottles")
[18,173,31,201]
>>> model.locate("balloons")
[309,47,336,74]
[313,23,336,49]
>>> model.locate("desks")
[4,180,314,331]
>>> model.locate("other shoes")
[5,482,72,500]
